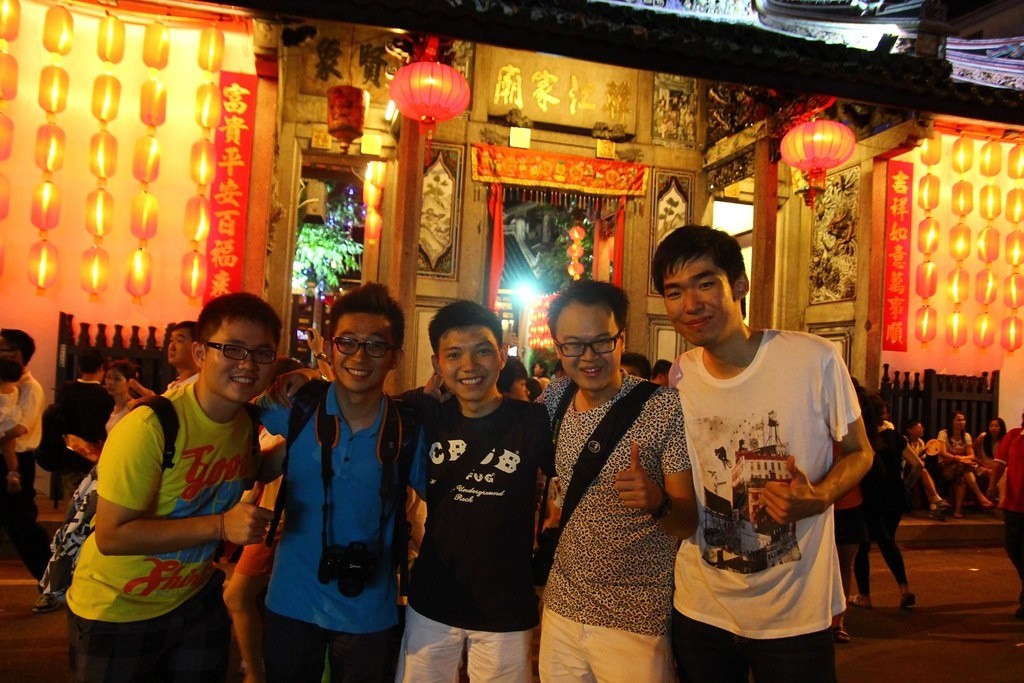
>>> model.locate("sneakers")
[32,594,61,612]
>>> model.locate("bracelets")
[314,352,329,362]
[221,511,227,541]
[319,372,327,381]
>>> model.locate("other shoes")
[931,499,952,511]
[928,511,946,522]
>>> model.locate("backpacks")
[38,394,181,603]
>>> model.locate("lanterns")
[779,120,856,187]
[566,226,586,281]
[363,161,386,245]
[529,295,555,348]
[1,0,226,298]
[390,62,470,135]
[327,85,363,154]
[915,131,1024,352]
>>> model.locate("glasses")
[206,341,276,364]
[552,326,624,358]
[332,336,397,358]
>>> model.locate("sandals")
[833,626,850,643]
[900,593,915,607]
[848,595,871,608]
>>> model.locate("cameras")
[319,541,382,598]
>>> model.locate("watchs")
[649,489,672,519]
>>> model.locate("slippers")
[979,498,995,509]
[953,511,963,519]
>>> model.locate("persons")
[127,282,428,683]
[986,414,1024,619]
[533,279,699,683]
[497,352,672,549]
[652,223,876,683]
[67,291,286,682]
[396,298,557,683]
[0,320,200,612]
[828,376,1007,644]
